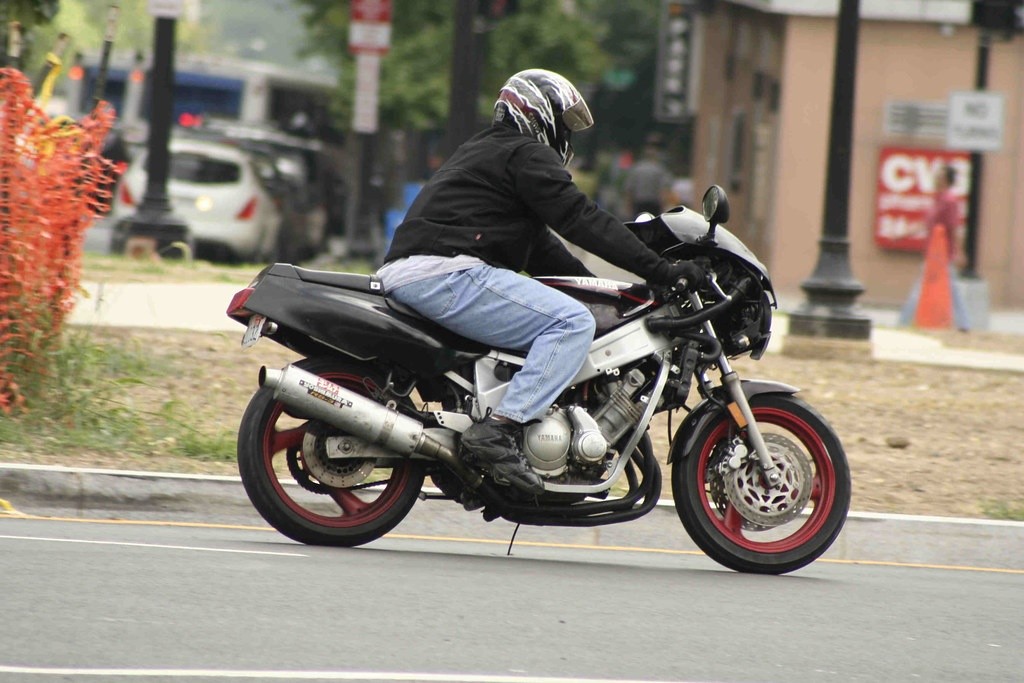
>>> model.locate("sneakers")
[462,407,545,495]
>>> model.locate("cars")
[112,143,282,266]
[191,123,341,262]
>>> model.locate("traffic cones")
[912,221,955,330]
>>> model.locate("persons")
[376,67,708,501]
[623,143,673,227]
[900,166,972,333]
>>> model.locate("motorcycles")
[227,187,855,578]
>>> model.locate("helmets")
[491,69,594,171]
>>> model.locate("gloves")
[661,261,709,294]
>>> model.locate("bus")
[63,52,131,154]
[122,48,360,196]
